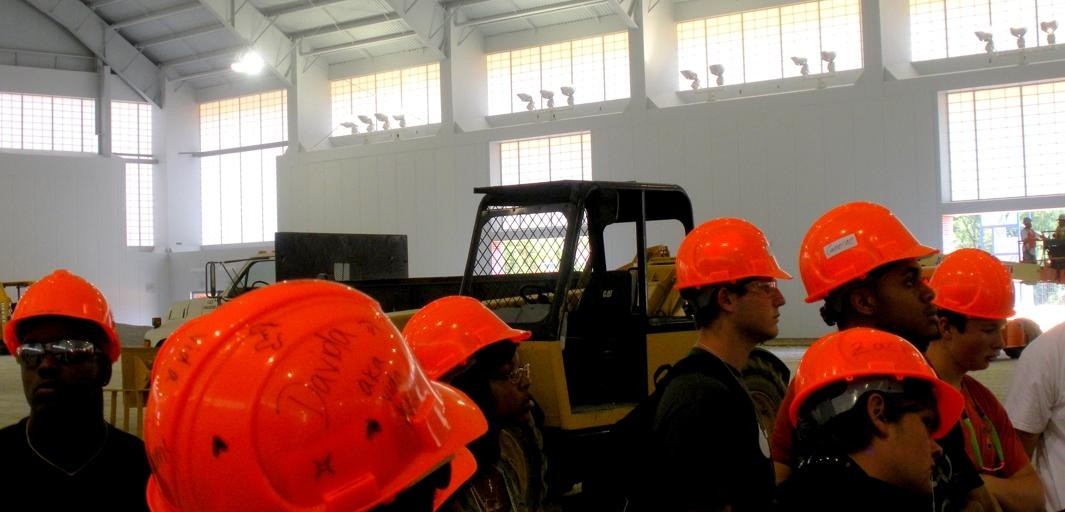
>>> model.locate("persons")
[402,293,556,511]
[1052,213,1065,240]
[924,248,1047,512]
[1,269,149,511]
[1003,321,1065,511]
[642,216,783,508]
[1021,217,1044,264]
[773,324,967,508]
[797,198,1001,512]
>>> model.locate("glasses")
[727,278,778,298]
[16,338,99,373]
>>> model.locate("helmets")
[672,216,796,291]
[796,199,943,306]
[786,326,969,442]
[1,267,124,366]
[399,295,533,384]
[927,247,1018,322]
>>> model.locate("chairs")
[564,270,633,388]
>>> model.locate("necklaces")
[22,414,84,484]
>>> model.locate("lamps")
[517,85,577,111]
[679,64,725,91]
[975,20,1059,64]
[791,51,837,80]
[340,112,406,136]
